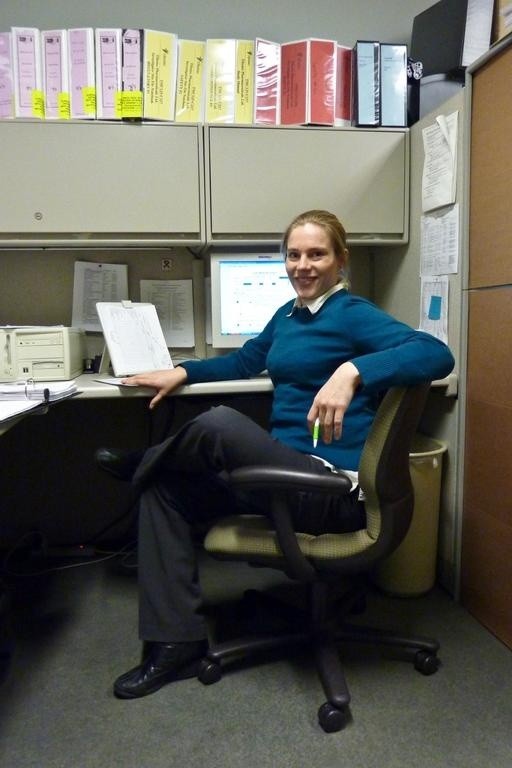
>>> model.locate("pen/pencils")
[312,416,319,448]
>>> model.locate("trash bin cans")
[375,436,448,598]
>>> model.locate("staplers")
[83,359,93,374]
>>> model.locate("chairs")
[197,375,442,734]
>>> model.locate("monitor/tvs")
[209,252,299,348]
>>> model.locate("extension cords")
[31,544,98,557]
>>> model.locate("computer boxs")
[0,326,89,383]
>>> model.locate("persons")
[90,208,456,701]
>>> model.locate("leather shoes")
[95,447,146,483]
[110,637,211,700]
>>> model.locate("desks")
[1,368,461,598]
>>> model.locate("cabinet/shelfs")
[202,122,413,250]
[1,119,206,253]
